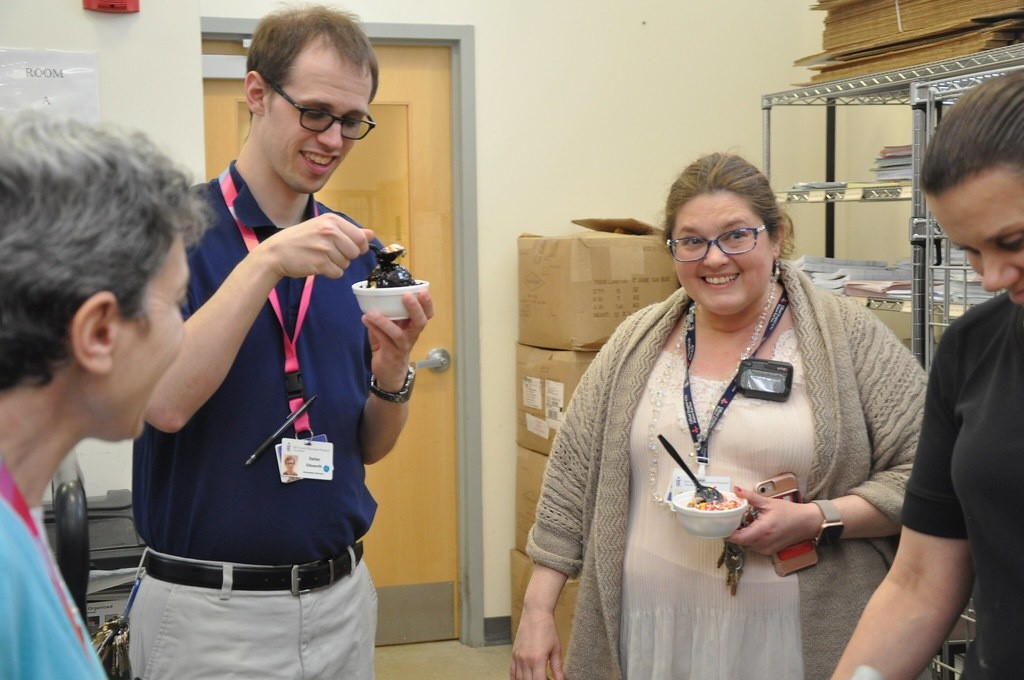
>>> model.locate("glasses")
[264,71,375,141]
[667,221,766,264]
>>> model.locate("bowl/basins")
[353,279,430,321]
[673,491,747,540]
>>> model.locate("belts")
[144,542,365,590]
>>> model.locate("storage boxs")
[509,215,682,680]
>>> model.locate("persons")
[0,108,200,680]
[508,156,927,680]
[831,66,1024,680]
[126,5,433,680]
[284,456,297,476]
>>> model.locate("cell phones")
[754,472,819,577]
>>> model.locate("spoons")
[368,242,405,262]
[656,434,724,502]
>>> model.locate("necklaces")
[647,277,776,506]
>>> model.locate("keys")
[91,618,129,677]
[717,542,745,595]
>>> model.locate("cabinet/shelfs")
[759,42,1024,680]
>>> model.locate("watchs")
[369,366,416,403]
[812,500,844,547]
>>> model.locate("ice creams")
[365,244,417,288]
